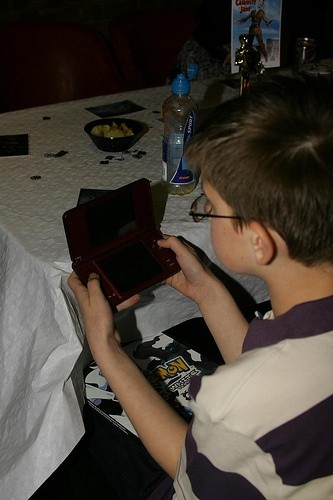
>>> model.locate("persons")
[237,1,273,65]
[236,35,264,92]
[67,77,333,500]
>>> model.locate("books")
[80,332,202,444]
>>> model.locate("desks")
[0,65,333,499]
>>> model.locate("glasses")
[189,194,244,223]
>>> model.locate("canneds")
[292,36,315,67]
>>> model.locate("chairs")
[111,8,212,91]
[12,21,125,106]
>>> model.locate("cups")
[294,38,316,66]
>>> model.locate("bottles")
[160,63,198,195]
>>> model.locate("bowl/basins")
[83,117,150,153]
